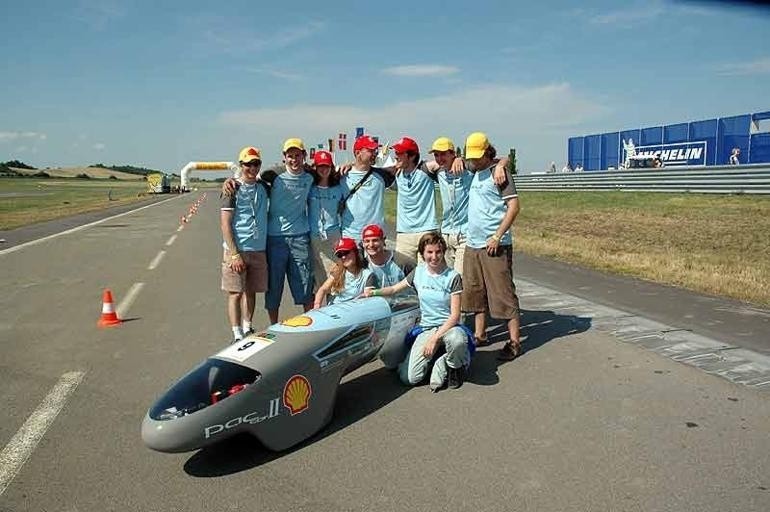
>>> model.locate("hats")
[315,152,332,167]
[282,138,305,152]
[465,133,489,159]
[333,239,357,255]
[353,136,382,151]
[239,147,262,163]
[362,225,384,240]
[428,138,455,154]
[389,138,419,153]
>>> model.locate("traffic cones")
[94,286,123,328]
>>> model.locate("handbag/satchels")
[338,168,375,213]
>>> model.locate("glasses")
[336,251,351,258]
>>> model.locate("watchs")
[491,234,501,241]
[231,253,241,260]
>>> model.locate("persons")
[562,164,573,172]
[339,133,398,262]
[221,137,314,329]
[314,237,378,310]
[574,163,584,172]
[339,136,465,268]
[461,131,523,362]
[217,145,270,346]
[653,154,665,167]
[729,147,741,166]
[364,229,472,390]
[328,223,417,294]
[427,136,510,324]
[307,150,345,311]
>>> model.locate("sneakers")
[234,337,243,344]
[497,342,523,361]
[447,367,464,389]
[472,336,489,347]
[244,329,253,336]
